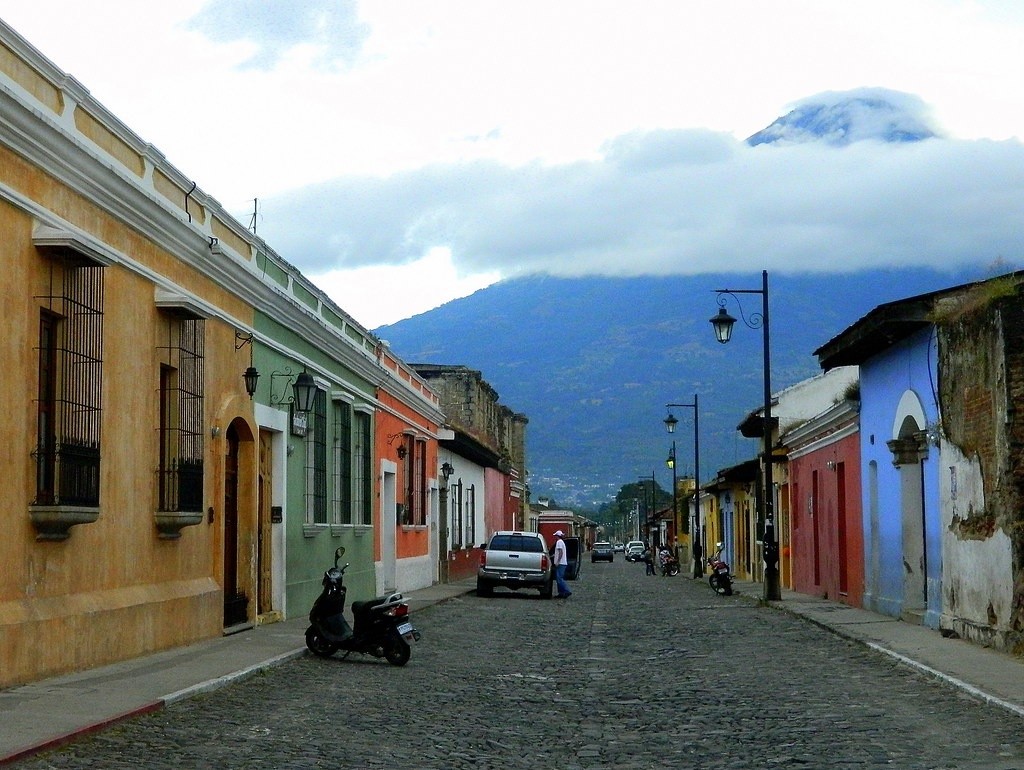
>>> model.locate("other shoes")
[653,574,657,575]
[554,592,572,600]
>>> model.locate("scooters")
[304,548,420,666]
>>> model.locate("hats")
[553,531,564,536]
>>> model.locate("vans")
[623,541,645,558]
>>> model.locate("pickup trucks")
[614,544,624,554]
[475,532,581,599]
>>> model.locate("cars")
[592,542,613,563]
[628,547,647,560]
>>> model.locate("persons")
[551,531,572,598]
[645,545,656,575]
[657,542,673,576]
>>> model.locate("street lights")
[667,441,678,563]
[638,476,657,560]
[663,394,702,581]
[710,288,783,600]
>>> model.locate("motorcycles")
[665,557,678,576]
[701,542,736,596]
